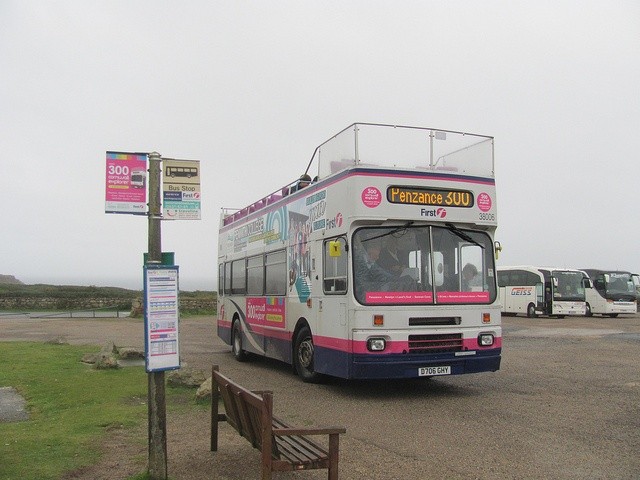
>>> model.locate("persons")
[288,217,312,282]
[286,174,311,195]
[369,243,398,282]
[441,264,477,292]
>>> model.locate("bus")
[216,122,503,384]
[581,269,639,317]
[497,267,593,318]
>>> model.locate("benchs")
[210,365,346,480]
